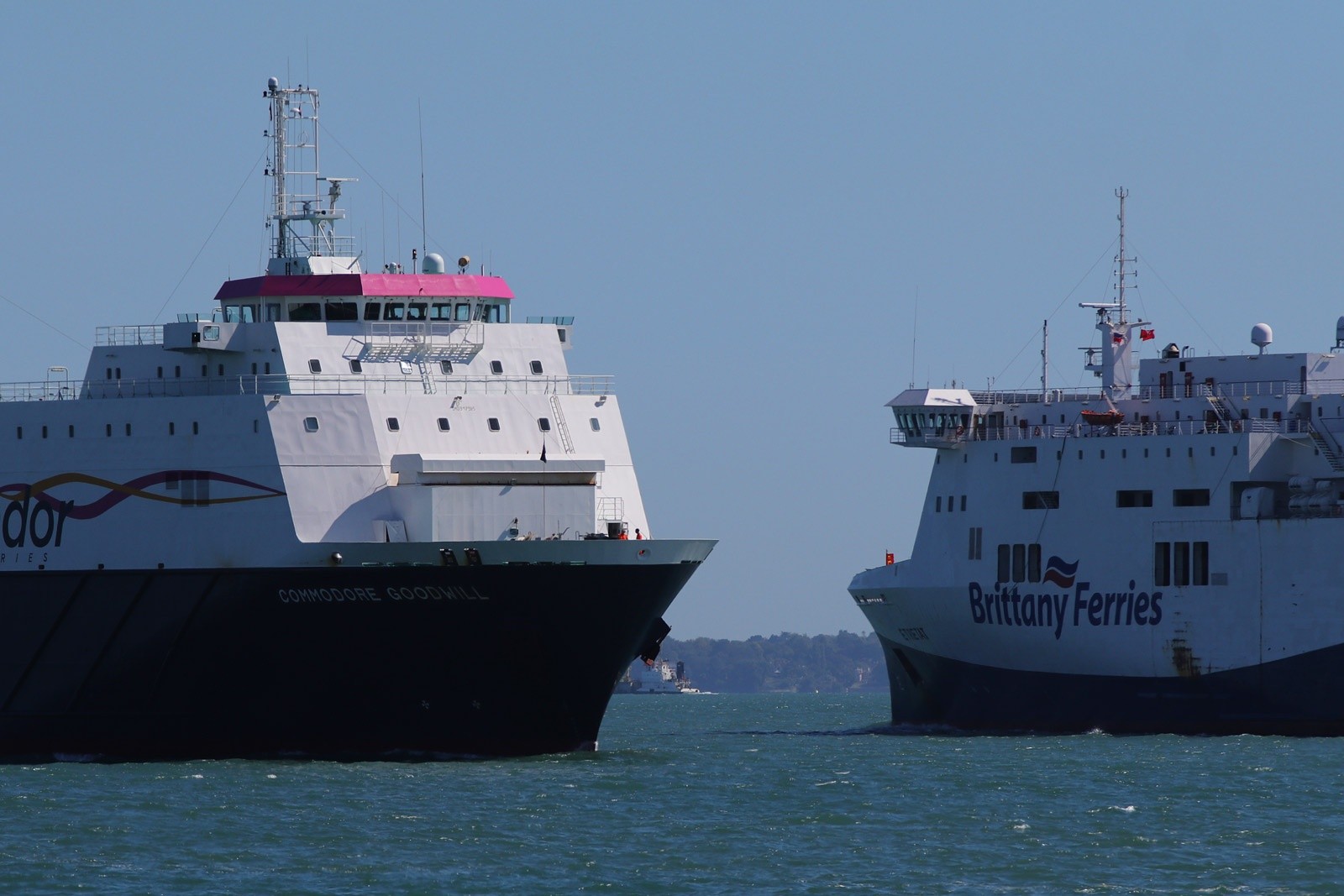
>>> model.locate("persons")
[635,528,642,539]
[618,530,628,540]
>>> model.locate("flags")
[1140,329,1155,341]
[1109,329,1128,348]
[886,553,894,565]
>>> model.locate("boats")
[843,185,1344,729]
[1080,410,1125,426]
[635,659,700,693]
[1,80,718,752]
